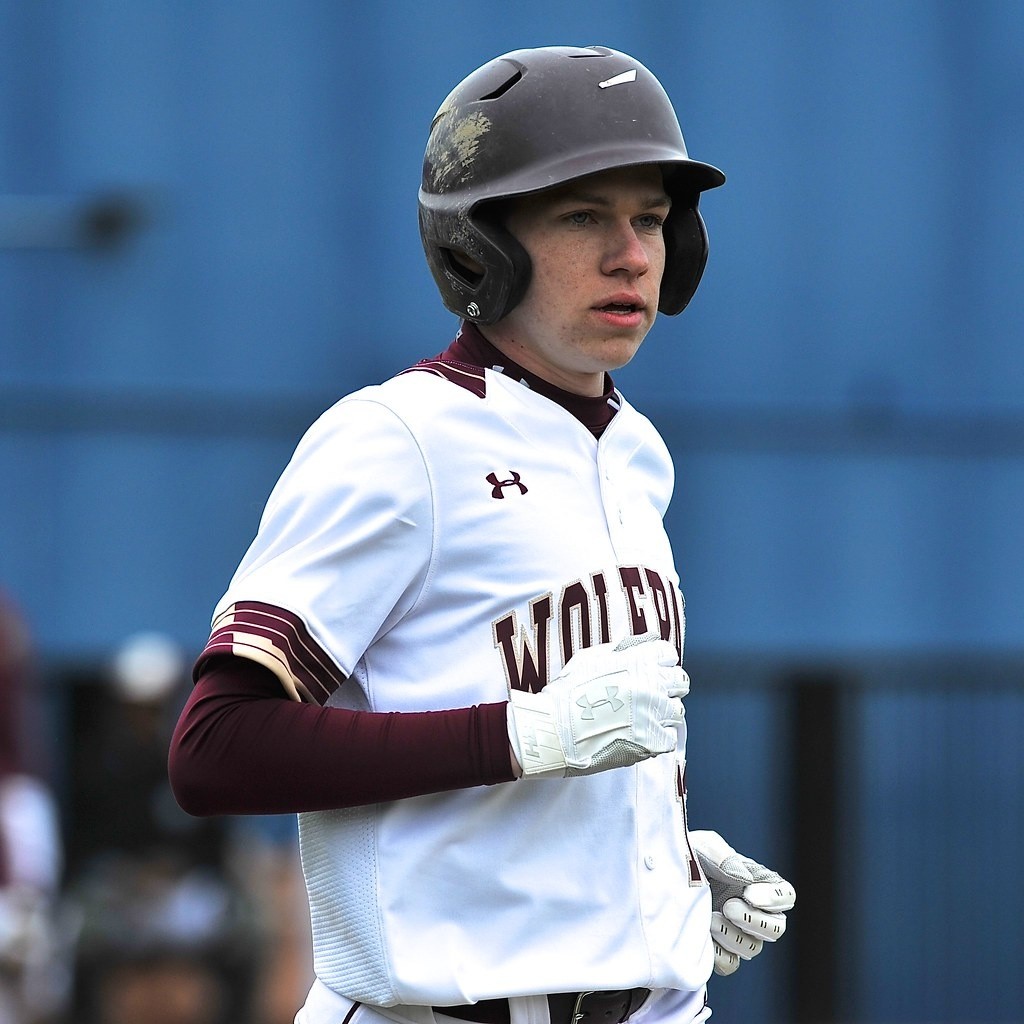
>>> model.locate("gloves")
[689,830,796,977]
[507,632,691,781]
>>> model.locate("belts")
[431,987,651,1024]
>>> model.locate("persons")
[166,37,804,1024]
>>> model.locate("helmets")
[419,45,726,324]
